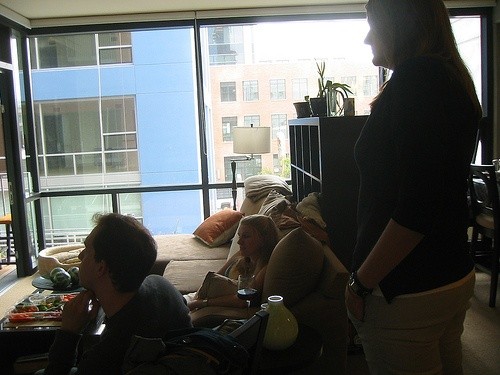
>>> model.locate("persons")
[187,215,281,311]
[34,213,193,375]
[345,0,481,375]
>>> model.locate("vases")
[292,102,311,119]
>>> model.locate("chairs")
[468,163,500,307]
[215,311,270,375]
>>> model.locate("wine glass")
[237,274,259,323]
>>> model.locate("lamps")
[228,122,271,162]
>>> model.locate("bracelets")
[203,297,208,307]
[347,272,372,298]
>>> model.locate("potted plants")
[310,60,353,117]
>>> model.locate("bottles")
[262,295,298,351]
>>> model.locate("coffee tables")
[0,288,82,375]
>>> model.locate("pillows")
[260,227,322,309]
[194,209,246,249]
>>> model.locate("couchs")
[39,244,88,278]
[150,195,350,328]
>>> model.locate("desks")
[287,115,370,252]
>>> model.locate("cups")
[344,98,354,116]
